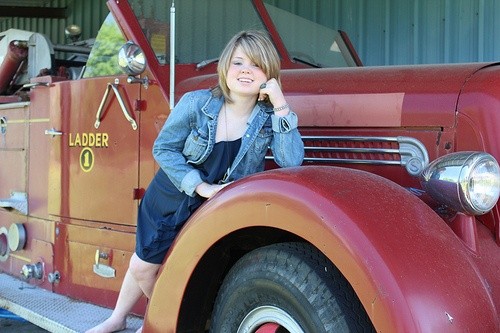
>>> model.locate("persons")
[81,30,306,333]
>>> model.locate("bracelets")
[272,102,290,113]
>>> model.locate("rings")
[259,83,267,89]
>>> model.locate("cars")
[0,0,500,333]
